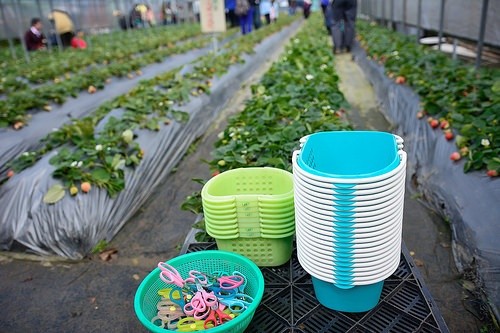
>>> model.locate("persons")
[118,1,200,31]
[224,0,279,36]
[288,0,297,15]
[303,0,312,19]
[321,0,357,55]
[24,6,87,54]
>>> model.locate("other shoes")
[334,46,349,54]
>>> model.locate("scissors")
[152,262,253,331]
[204,272,243,289]
[191,278,216,312]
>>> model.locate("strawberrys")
[0,32,500,196]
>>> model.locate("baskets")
[134,249,265,333]
[200,166,295,268]
[293,130,407,312]
